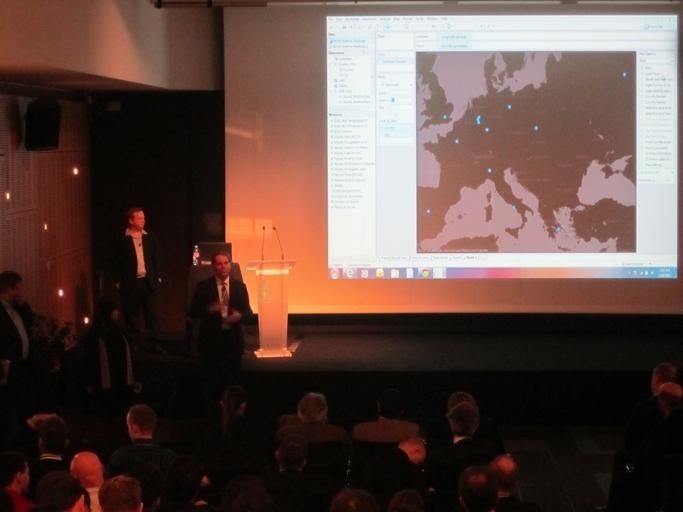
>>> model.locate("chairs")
[270,424,425,500]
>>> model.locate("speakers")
[24,97,61,151]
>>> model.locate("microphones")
[272,227,284,260]
[261,226,265,261]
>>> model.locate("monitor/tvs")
[199,242,232,263]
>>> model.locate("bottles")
[192,245,199,266]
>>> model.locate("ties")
[221,281,229,307]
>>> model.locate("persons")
[108,203,168,355]
[619,359,681,461]
[653,383,682,436]
[1,383,548,512]
[0,268,37,452]
[78,299,144,441]
[15,287,74,384]
[188,251,252,420]
